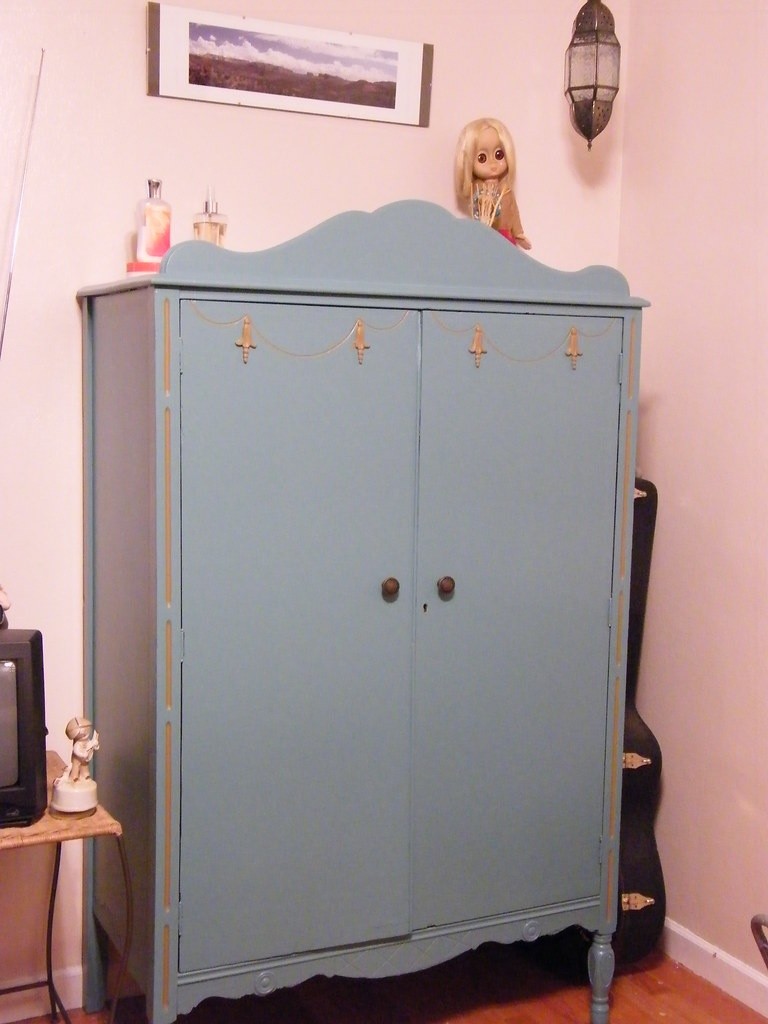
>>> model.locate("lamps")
[564,0,621,150]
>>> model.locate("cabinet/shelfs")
[79,202,652,1024]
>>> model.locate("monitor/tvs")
[0,628,48,829]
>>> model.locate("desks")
[0,751,121,1024]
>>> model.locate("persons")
[66,716,99,785]
[452,117,532,252]
